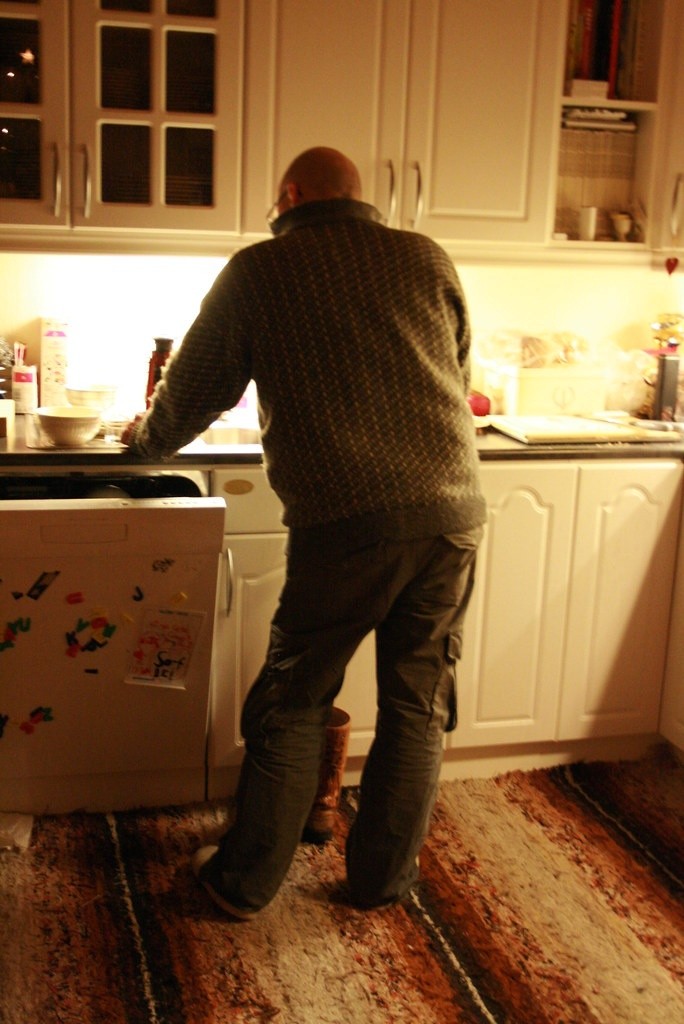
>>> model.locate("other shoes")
[191,844,258,921]
[266,189,300,222]
[372,855,419,911]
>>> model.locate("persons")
[118,146,488,920]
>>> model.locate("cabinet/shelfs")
[244,0,684,260]
[1,0,244,259]
[441,460,684,775]
[207,468,378,795]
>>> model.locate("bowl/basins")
[35,405,103,446]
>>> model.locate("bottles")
[144,337,175,415]
